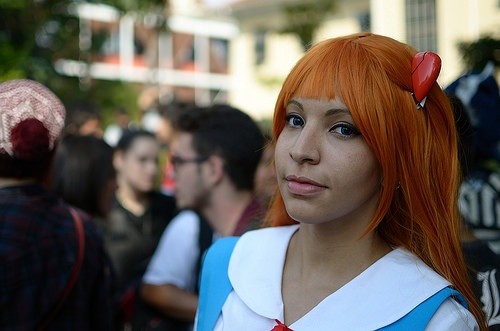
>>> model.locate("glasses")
[170,154,209,168]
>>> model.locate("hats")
[0,79,65,160]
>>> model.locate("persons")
[1,80,499,331]
[442,40,500,174]
[192,32,488,331]
